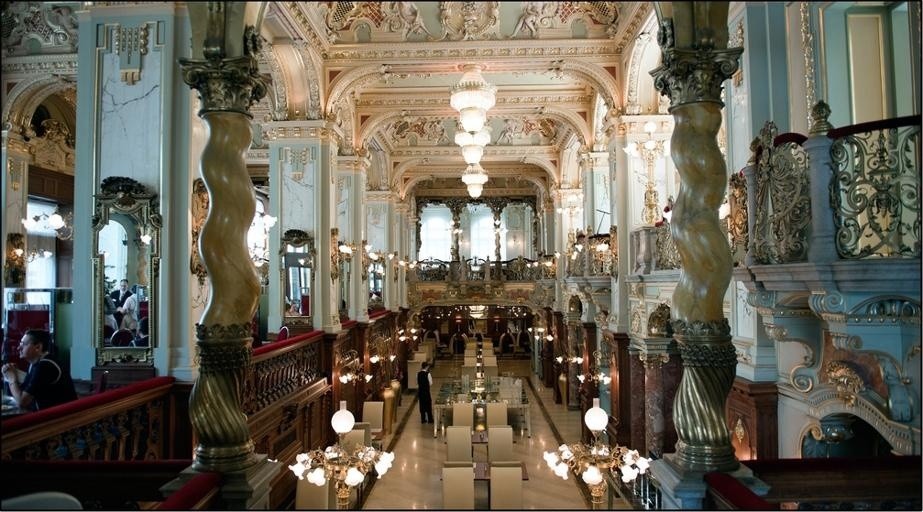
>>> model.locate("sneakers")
[421,419,434,424]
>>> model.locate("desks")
[440,462,529,481]
[432,377,532,439]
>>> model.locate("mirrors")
[280,229,385,329]
[90,175,163,367]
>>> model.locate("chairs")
[442,330,523,510]
[407,329,447,395]
[76,371,107,400]
[296,401,384,509]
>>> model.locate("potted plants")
[371,328,402,434]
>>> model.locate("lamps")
[3,232,53,304]
[449,63,498,201]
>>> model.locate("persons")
[469,318,475,330]
[103,278,148,348]
[285,295,302,317]
[414,361,433,424]
[0,329,78,458]
[369,288,381,303]
